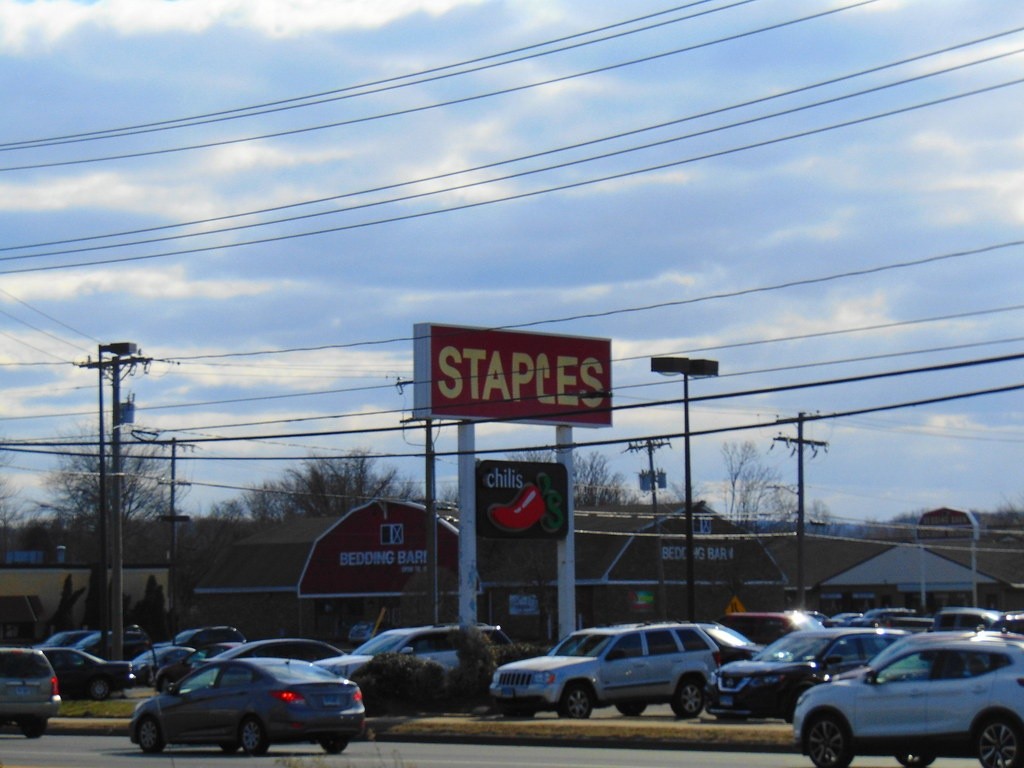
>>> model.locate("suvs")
[309,621,523,689]
[790,625,1023,768]
[487,622,725,719]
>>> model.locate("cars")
[190,638,349,675]
[0,647,63,739]
[149,624,249,651]
[25,627,150,658]
[153,641,246,695]
[130,647,196,687]
[719,605,1024,646]
[128,657,367,756]
[702,628,915,724]
[42,647,135,701]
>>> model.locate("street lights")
[650,355,718,617]
